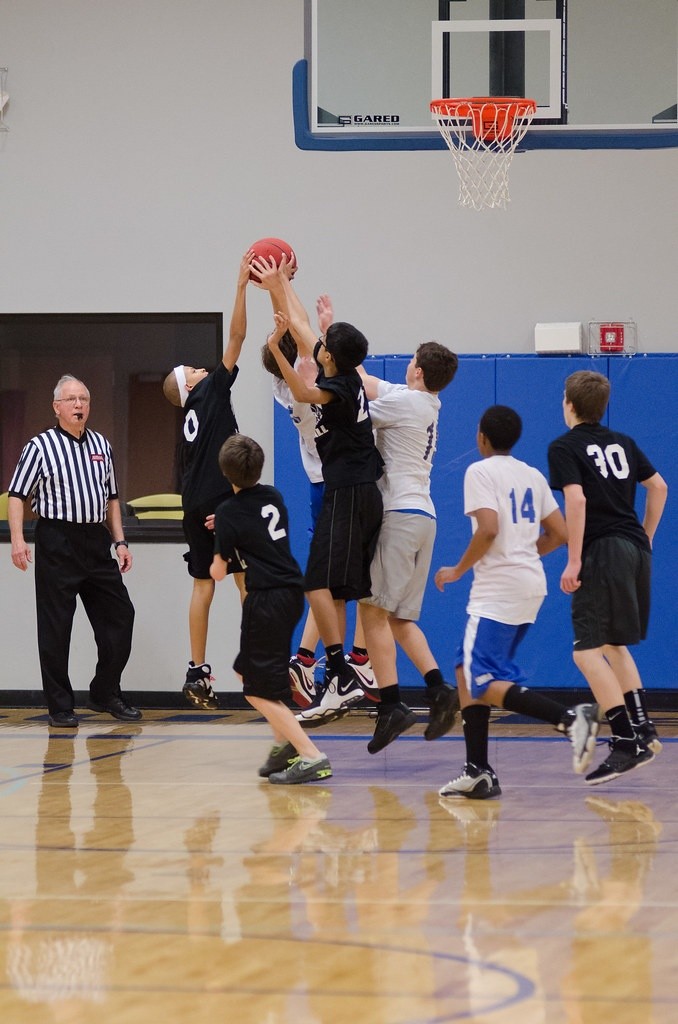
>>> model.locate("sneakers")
[183,660,220,710]
[438,762,501,799]
[584,795,662,846]
[367,700,417,754]
[371,784,418,831]
[260,782,333,820]
[345,651,383,702]
[294,667,365,728]
[259,741,300,777]
[554,702,603,775]
[634,720,663,754]
[440,799,502,828]
[268,753,332,785]
[424,792,468,852]
[287,654,317,707]
[584,726,655,786]
[424,682,460,740]
[182,810,222,857]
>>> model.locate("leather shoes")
[86,690,142,720]
[47,709,78,727]
[87,725,143,758]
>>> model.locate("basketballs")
[245,238,298,290]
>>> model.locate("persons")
[162,245,256,712]
[246,251,460,754]
[6,373,142,729]
[433,403,604,800]
[545,369,669,787]
[204,432,335,785]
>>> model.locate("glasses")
[57,396,90,403]
[319,336,334,363]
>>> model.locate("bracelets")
[114,540,128,550]
